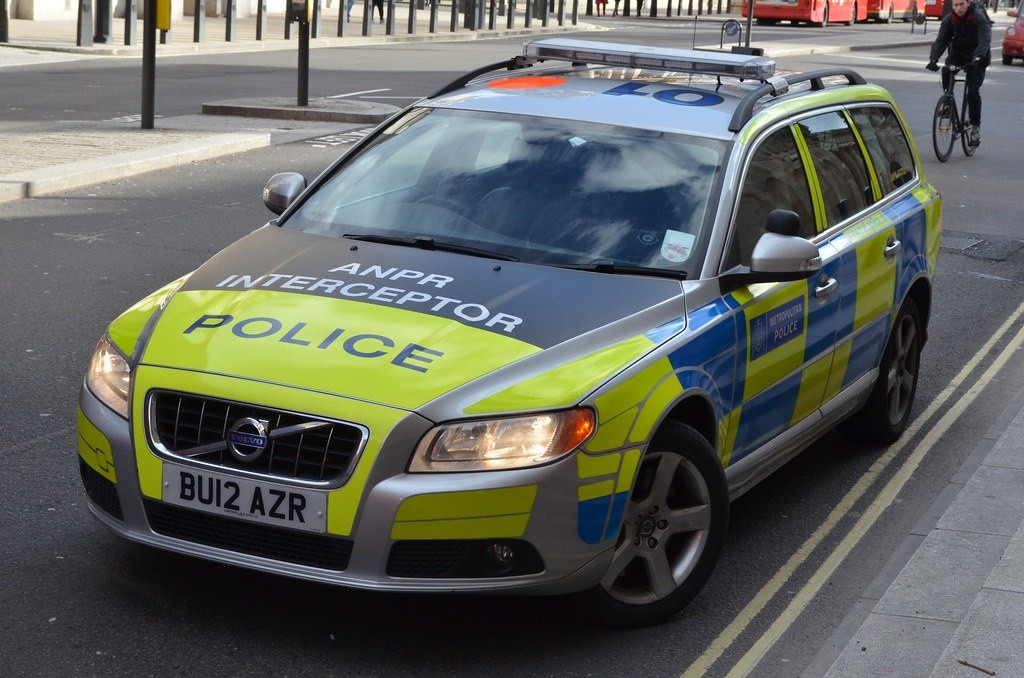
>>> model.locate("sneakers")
[970,126,980,142]
[939,108,951,117]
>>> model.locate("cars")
[1002,0,1024,65]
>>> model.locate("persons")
[926,0,995,148]
[890,153,914,187]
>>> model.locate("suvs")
[74,33,944,626]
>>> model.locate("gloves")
[965,61,979,72]
[926,63,940,72]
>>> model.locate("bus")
[925,0,947,21]
[867,0,926,25]
[742,0,869,28]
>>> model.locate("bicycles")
[932,62,978,163]
